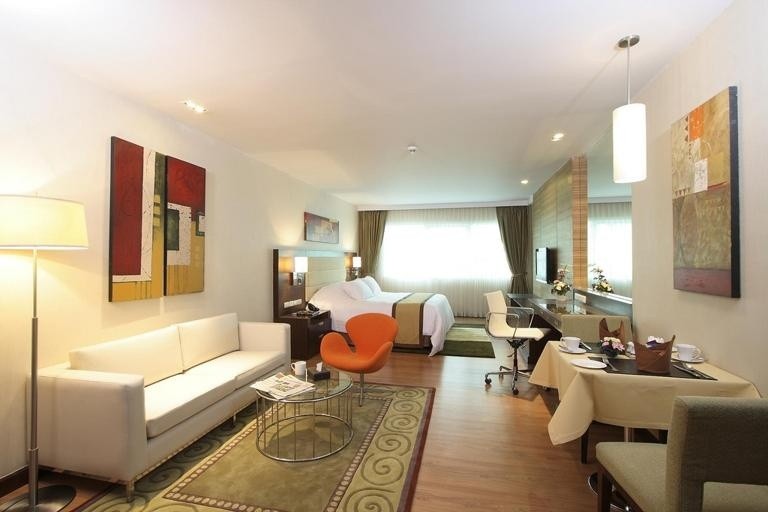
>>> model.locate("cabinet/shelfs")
[505,290,550,364]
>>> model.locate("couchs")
[22,310,295,502]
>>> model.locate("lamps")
[612,31,653,184]
[281,255,310,287]
[343,255,362,275]
[0,190,95,512]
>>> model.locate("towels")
[598,317,626,346]
[632,332,678,373]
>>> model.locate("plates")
[559,347,586,354]
[672,348,677,353]
[671,356,704,362]
[571,359,608,369]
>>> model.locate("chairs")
[482,287,552,396]
[318,312,399,416]
[558,309,638,465]
[594,387,768,511]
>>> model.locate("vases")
[555,293,568,310]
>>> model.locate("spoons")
[682,363,710,379]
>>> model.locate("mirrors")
[585,124,636,310]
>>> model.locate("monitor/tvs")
[535,247,550,284]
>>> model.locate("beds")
[305,253,457,356]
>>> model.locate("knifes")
[672,364,701,377]
[580,342,592,350]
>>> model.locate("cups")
[626,342,633,353]
[560,336,578,352]
[290,360,307,376]
[677,344,701,361]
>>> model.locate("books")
[249,371,316,400]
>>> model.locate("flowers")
[551,265,570,295]
[587,262,618,293]
[598,334,624,355]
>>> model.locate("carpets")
[71,369,438,512]
[388,321,497,358]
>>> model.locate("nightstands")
[280,307,333,361]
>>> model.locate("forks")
[603,359,619,371]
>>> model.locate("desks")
[527,296,634,344]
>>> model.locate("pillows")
[342,276,374,299]
[362,275,382,296]
